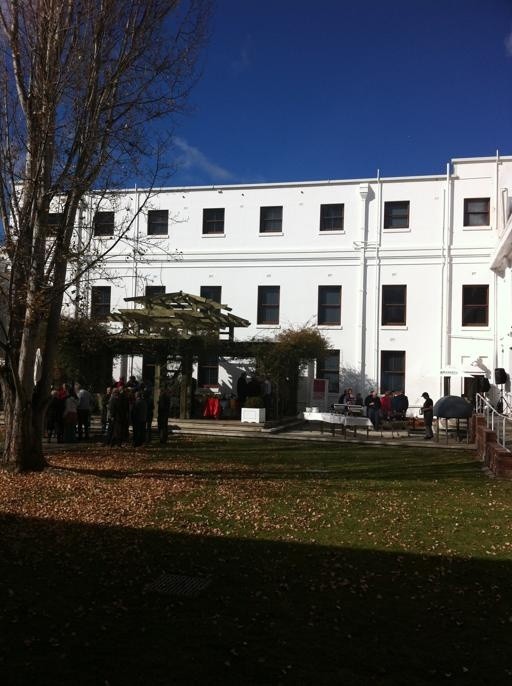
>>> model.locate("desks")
[303,412,369,440]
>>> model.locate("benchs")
[381,414,415,439]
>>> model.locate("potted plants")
[241,397,266,423]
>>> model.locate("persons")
[238,372,247,403]
[342,388,408,431]
[43,374,173,449]
[421,393,433,439]
